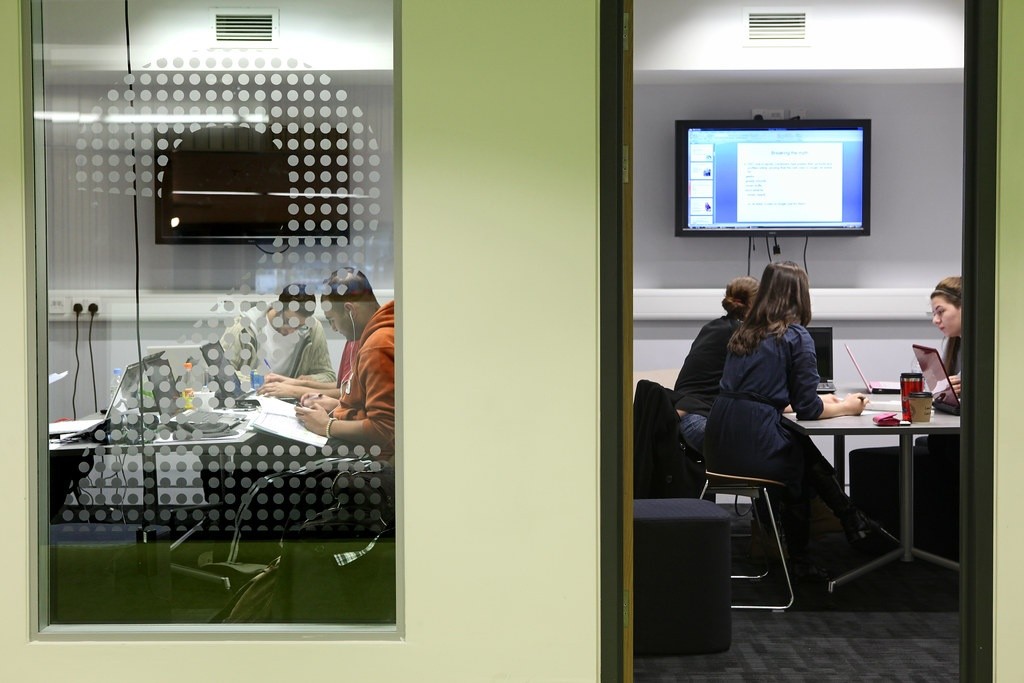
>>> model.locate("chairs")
[282,439,397,535]
[636,379,794,613]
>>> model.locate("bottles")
[109,369,125,411]
[181,363,194,409]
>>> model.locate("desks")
[781,388,961,593]
[49,396,281,547]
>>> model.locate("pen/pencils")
[51,440,79,443]
[299,394,324,405]
[222,407,255,412]
[857,396,866,400]
[264,358,272,374]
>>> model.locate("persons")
[702,259,879,598]
[672,277,847,568]
[866,277,961,566]
[220,283,336,384]
[256,341,359,400]
[232,267,394,564]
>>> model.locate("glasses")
[279,306,310,331]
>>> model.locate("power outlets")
[751,109,808,120]
[49,297,102,316]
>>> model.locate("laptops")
[143,351,247,430]
[912,344,961,416]
[64,361,147,441]
[807,326,836,394]
[200,339,297,406]
[845,344,902,394]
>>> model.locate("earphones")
[348,309,353,316]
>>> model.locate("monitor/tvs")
[675,119,872,238]
[153,125,351,245]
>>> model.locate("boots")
[749,532,788,566]
[805,495,844,539]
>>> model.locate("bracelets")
[326,418,336,440]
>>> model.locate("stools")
[634,498,733,656]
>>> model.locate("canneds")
[250,369,265,389]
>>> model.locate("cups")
[900,372,922,423]
[193,391,216,412]
[908,392,933,422]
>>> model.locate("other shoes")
[789,553,839,585]
[839,511,901,554]
